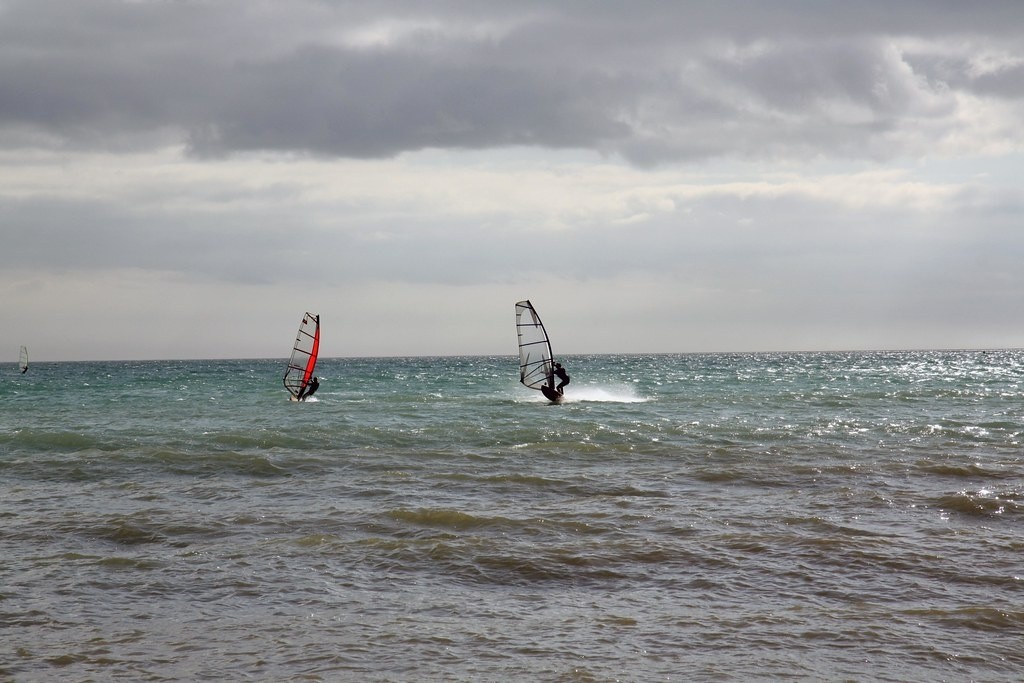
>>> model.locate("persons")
[300,376,320,401]
[552,360,571,395]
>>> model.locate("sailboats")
[515,299,563,401]
[283,311,320,403]
